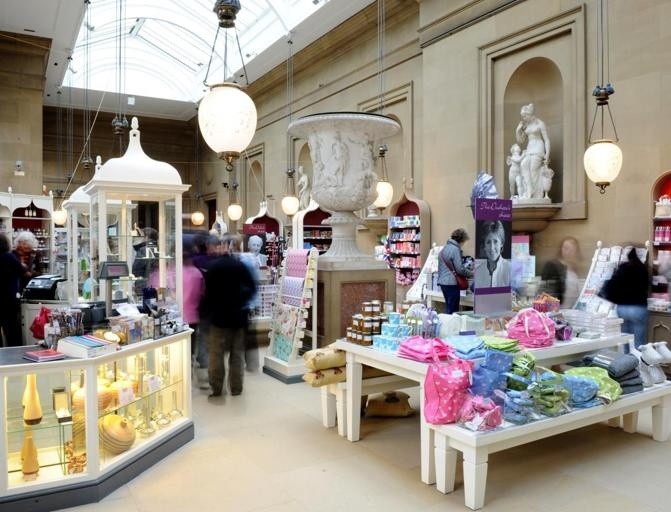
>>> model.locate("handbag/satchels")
[507,307,556,347]
[398,334,623,431]
[456,274,468,290]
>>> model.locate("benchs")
[426,377,670,510]
[320,375,420,437]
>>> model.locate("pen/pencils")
[52,312,82,336]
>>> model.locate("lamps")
[582,0,623,194]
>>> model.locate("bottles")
[20,431,40,475]
[20,373,45,425]
[346,299,387,347]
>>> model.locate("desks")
[334,331,635,485]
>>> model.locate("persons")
[596,243,652,350]
[131,227,256,399]
[0,233,33,347]
[476,220,511,289]
[294,163,312,211]
[503,140,523,201]
[246,235,266,268]
[437,228,476,314]
[308,127,374,189]
[516,102,557,206]
[537,236,582,308]
[12,228,44,283]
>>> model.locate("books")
[55,334,117,360]
[23,350,64,363]
[572,246,648,317]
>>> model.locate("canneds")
[345,298,395,345]
